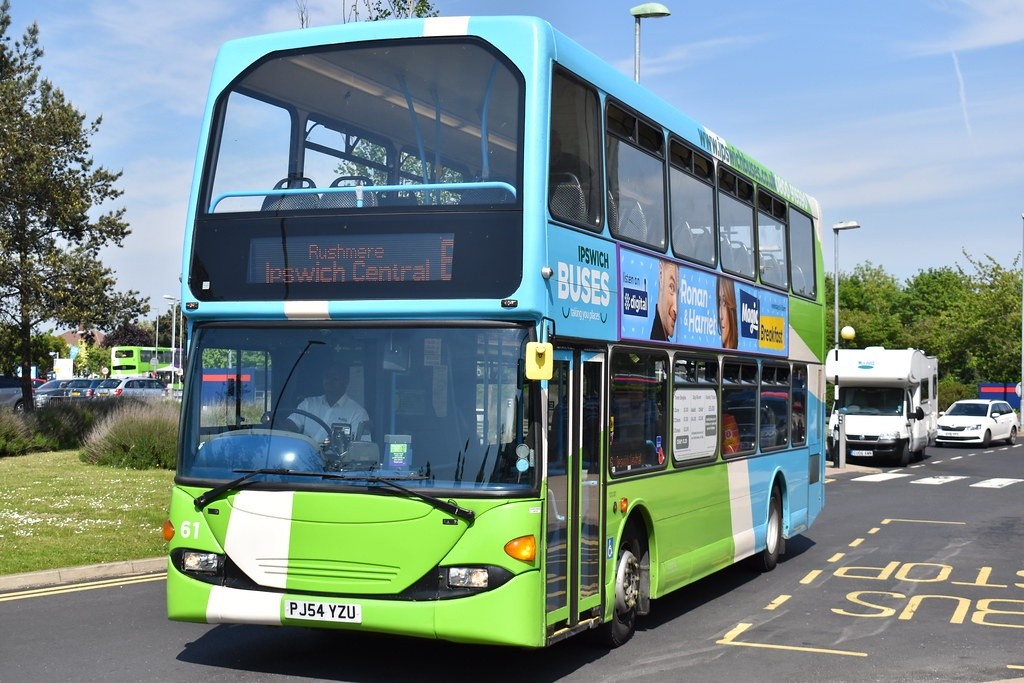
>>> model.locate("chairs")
[259,135,807,292]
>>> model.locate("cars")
[934,398,1019,449]
[30,378,106,409]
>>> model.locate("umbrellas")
[157,366,180,371]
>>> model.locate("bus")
[162,16,828,650]
[110,345,185,398]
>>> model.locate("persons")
[261,361,371,442]
[624,426,652,464]
[549,130,595,183]
[717,275,738,349]
[650,260,678,342]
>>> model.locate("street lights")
[629,2,671,83]
[163,294,178,399]
[832,220,861,467]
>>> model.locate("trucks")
[824,346,939,468]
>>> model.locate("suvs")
[0,374,33,416]
[92,377,167,400]
